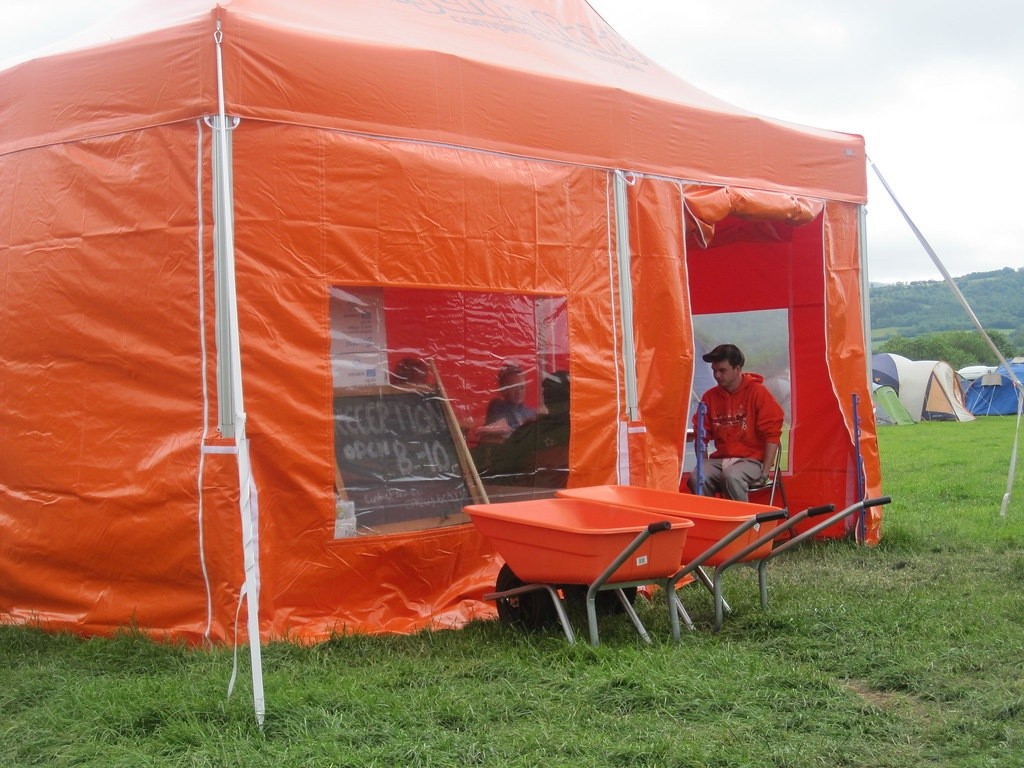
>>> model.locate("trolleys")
[458,484,892,653]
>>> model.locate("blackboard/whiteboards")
[330,384,478,543]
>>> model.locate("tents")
[871,353,976,425]
[954,357,1024,416]
[0,0,883,645]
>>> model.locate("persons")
[688,344,785,502]
[494,370,570,486]
[476,366,537,472]
[395,358,430,390]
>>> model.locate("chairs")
[716,443,795,538]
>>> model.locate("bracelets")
[759,476,769,483]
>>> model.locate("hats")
[702,344,745,366]
